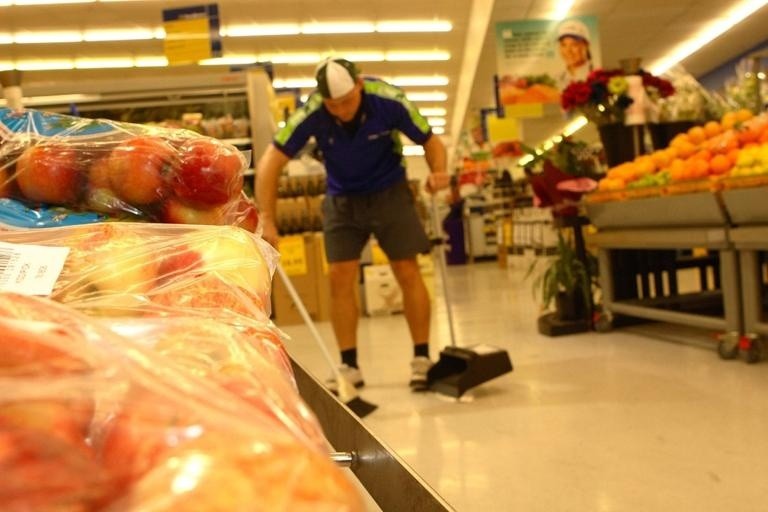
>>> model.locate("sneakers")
[409,355,434,390]
[322,364,365,394]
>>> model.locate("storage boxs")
[266,227,440,325]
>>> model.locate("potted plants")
[518,223,601,323]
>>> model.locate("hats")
[315,57,360,99]
[557,19,591,41]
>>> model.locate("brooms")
[276,261,380,420]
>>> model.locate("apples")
[0,129,280,251]
[0,223,377,512]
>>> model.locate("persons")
[555,19,595,85]
[254,58,453,394]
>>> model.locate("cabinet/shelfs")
[268,342,457,509]
[578,169,741,369]
[2,63,287,320]
[721,183,767,365]
